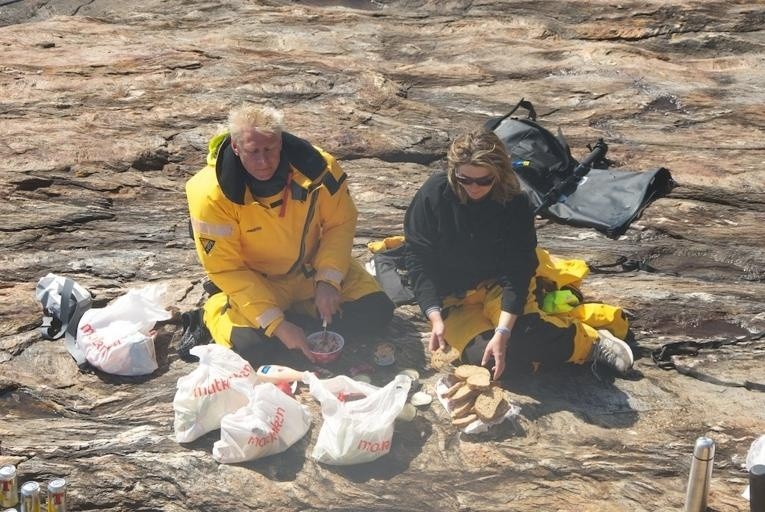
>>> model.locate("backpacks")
[482,97,680,240]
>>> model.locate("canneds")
[48,477,67,512]
[0,464,19,508]
[21,480,40,512]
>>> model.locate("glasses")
[455,172,495,186]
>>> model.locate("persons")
[403,126,636,382]
[184,101,398,369]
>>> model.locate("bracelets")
[493,327,512,338]
[262,316,283,339]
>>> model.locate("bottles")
[257,365,320,384]
[750,464,765,512]
[684,437,715,512]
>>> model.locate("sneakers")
[589,329,634,374]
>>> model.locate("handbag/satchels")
[36,272,92,340]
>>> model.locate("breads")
[430,345,462,373]
[442,365,509,428]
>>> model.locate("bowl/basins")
[306,331,345,363]
